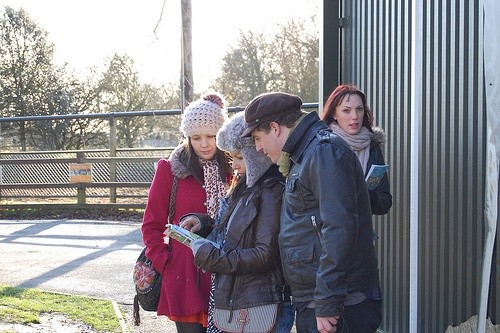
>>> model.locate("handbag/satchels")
[212,303,278,332]
[133,245,166,311]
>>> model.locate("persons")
[180,111,286,333]
[321,84,393,216]
[140,92,232,333]
[241,92,383,333]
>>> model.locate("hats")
[216,110,274,188]
[180,94,229,137]
[238,92,304,138]
